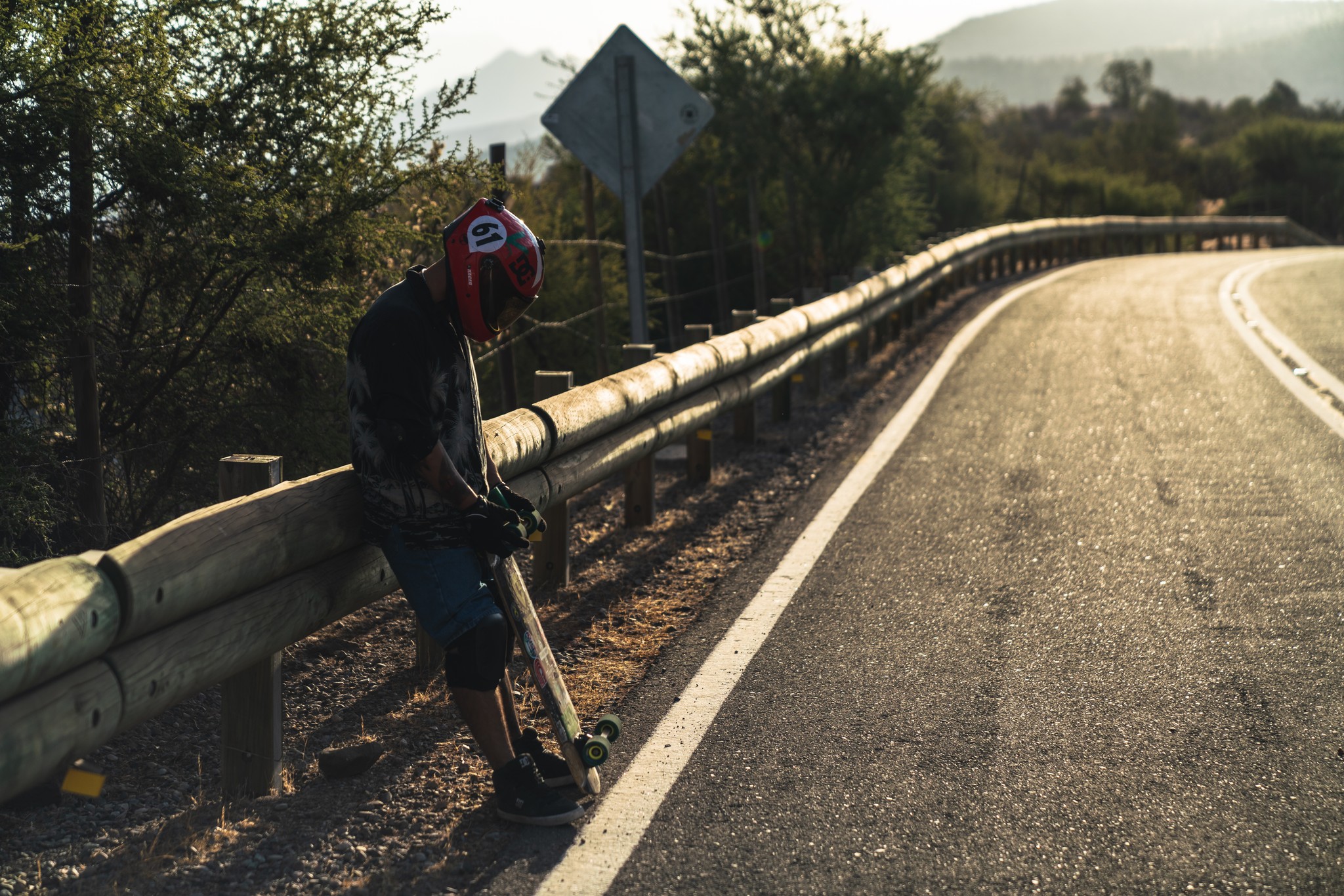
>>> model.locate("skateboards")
[478,485,624,796]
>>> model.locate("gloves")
[488,481,549,538]
[461,494,533,559]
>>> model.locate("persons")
[343,197,587,826]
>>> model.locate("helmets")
[442,197,549,341]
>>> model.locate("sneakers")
[511,726,575,788]
[492,751,585,826]
